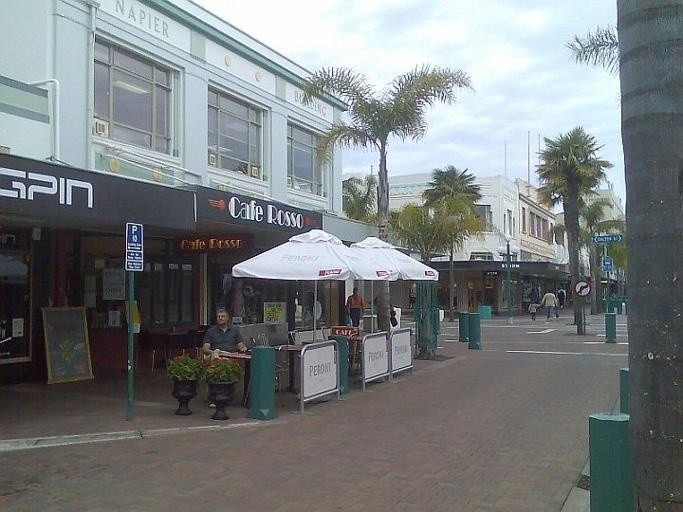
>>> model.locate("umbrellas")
[230,228,391,348]
[348,236,439,334]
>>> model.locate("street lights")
[504,234,512,317]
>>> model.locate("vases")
[171,380,236,420]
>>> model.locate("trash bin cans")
[478,305,491,319]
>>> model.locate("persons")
[527,288,565,321]
[408,287,415,309]
[373,289,394,330]
[201,307,248,408]
[344,288,366,328]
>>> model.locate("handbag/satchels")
[555,308,559,318]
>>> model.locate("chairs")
[244,326,362,410]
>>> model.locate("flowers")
[166,352,245,388]
